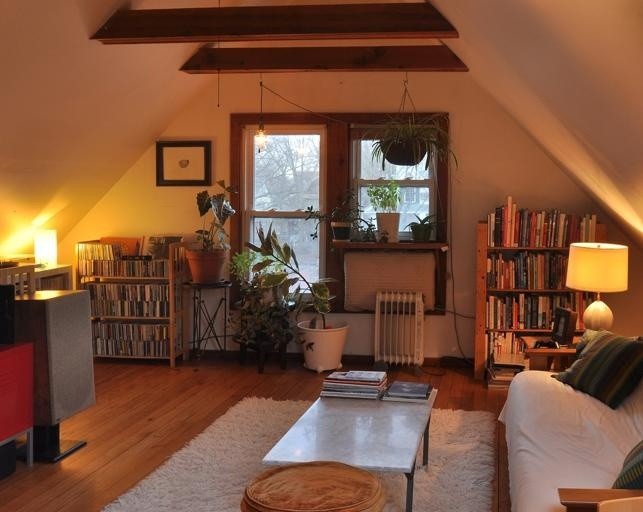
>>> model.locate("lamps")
[566,242,629,331]
[255,81,347,145]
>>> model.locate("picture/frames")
[156,139,211,187]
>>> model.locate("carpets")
[94,396,497,512]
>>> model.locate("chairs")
[1,283,34,467]
[1,265,36,294]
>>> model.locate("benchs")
[498,347,643,512]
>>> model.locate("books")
[486,291,592,330]
[485,331,530,388]
[487,194,597,248]
[78,240,180,279]
[78,282,182,318]
[487,249,569,291]
[91,322,182,357]
[318,370,434,404]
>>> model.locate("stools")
[241,461,385,512]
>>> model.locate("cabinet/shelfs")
[73,243,189,368]
[15,289,96,463]
[474,222,609,383]
[13,266,73,290]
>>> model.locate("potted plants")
[366,177,400,243]
[185,180,379,374]
[404,213,440,242]
[360,81,459,171]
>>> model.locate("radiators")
[373,290,425,376]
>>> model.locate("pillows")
[551,329,643,410]
[612,441,643,489]
